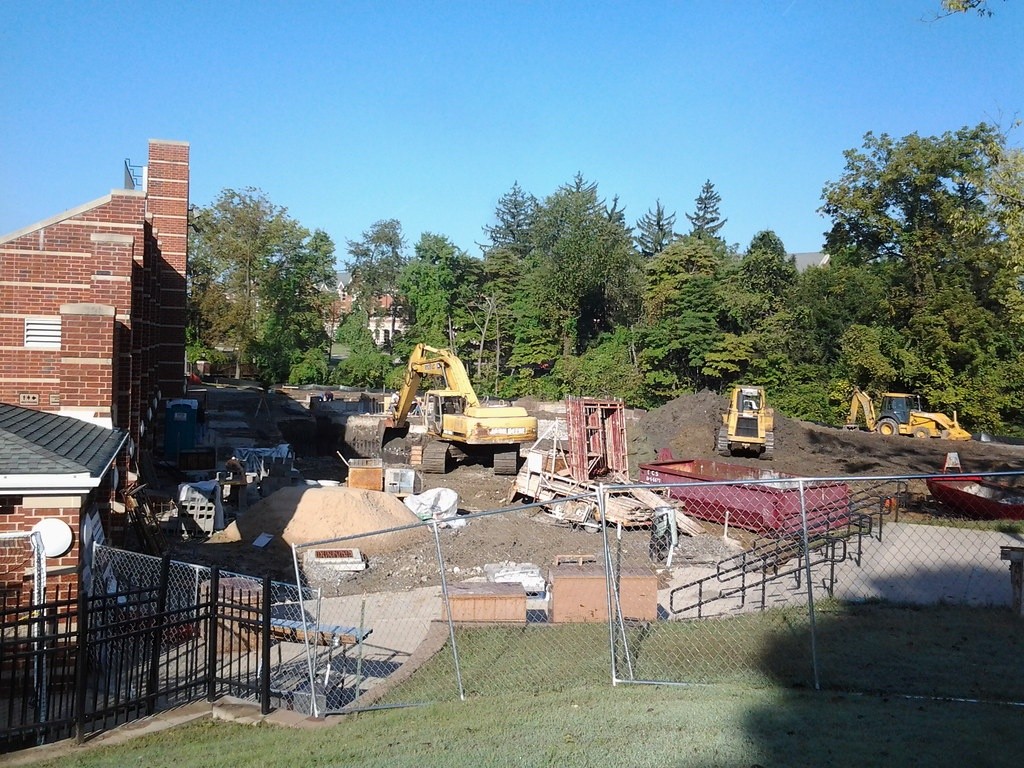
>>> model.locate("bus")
[719,385,777,461]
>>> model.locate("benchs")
[246,612,374,687]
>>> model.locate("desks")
[547,563,658,623]
[442,580,526,622]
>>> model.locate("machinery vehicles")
[377,340,538,477]
[844,386,973,441]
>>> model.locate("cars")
[183,369,319,445]
